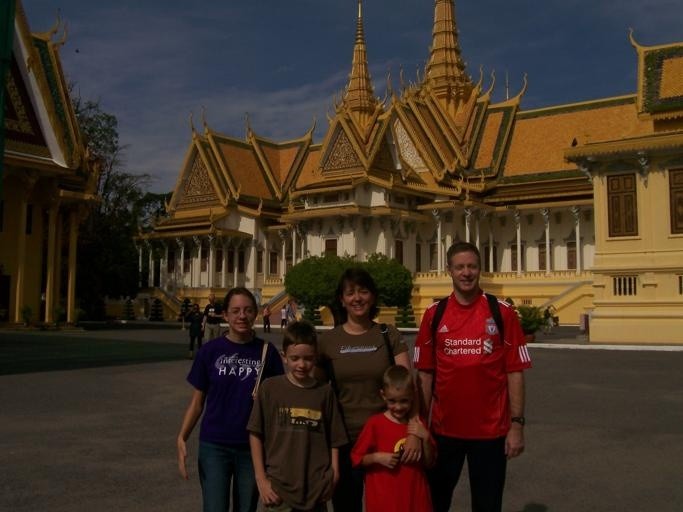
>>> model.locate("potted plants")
[517,305,549,343]
[20,303,34,328]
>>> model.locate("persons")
[309,267,422,512]
[413,242,531,512]
[350,365,438,512]
[281,300,296,329]
[262,304,271,333]
[246,322,347,512]
[179,287,284,512]
[185,293,224,360]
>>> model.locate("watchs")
[512,417,525,426]
